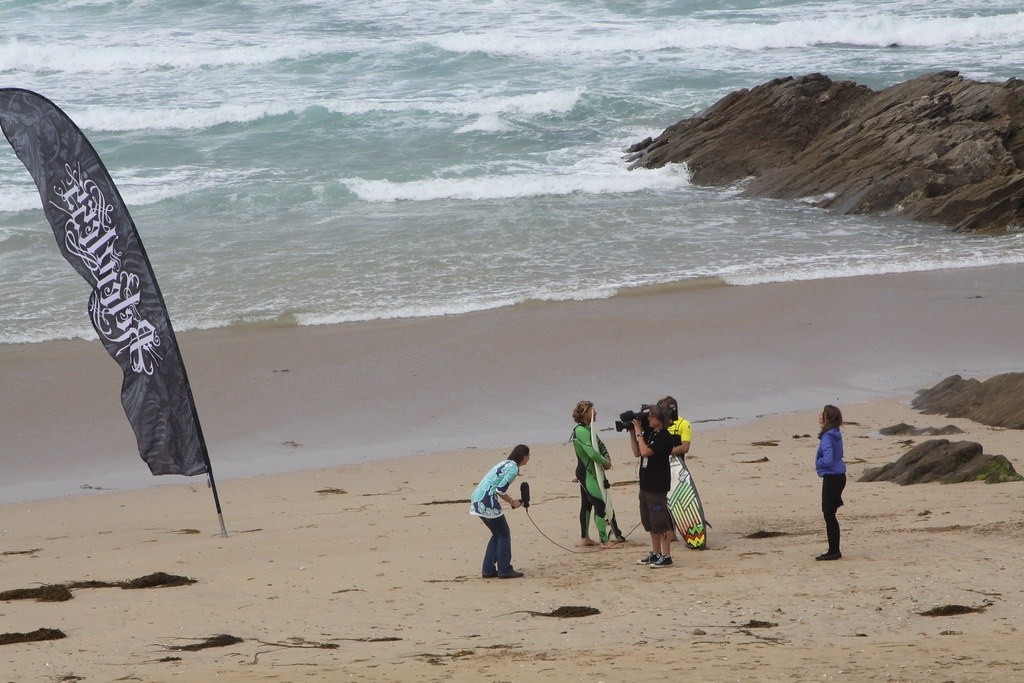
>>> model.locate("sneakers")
[649,555,673,568]
[637,552,662,564]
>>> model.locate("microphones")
[520,481,530,510]
[619,411,635,423]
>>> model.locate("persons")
[470,444,530,579]
[815,405,847,561]
[572,400,626,546]
[629,396,693,569]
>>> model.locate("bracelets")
[636,431,645,437]
[508,498,512,504]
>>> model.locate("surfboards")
[665,454,707,550]
[590,405,626,542]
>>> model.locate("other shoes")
[499,571,523,579]
[482,571,499,578]
[816,552,842,560]
